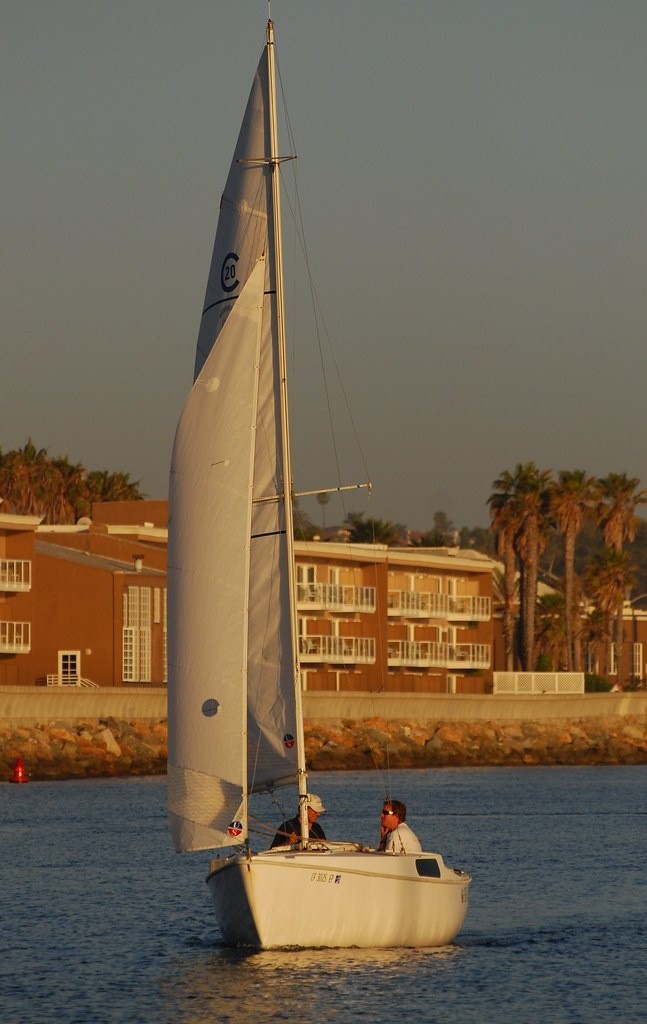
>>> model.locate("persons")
[269,794,327,850]
[378,800,422,854]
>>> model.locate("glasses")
[382,809,393,814]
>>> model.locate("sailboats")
[162,5,473,956]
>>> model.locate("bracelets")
[380,841,386,844]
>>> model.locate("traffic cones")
[9,755,30,785]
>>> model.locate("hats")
[298,793,327,812]
